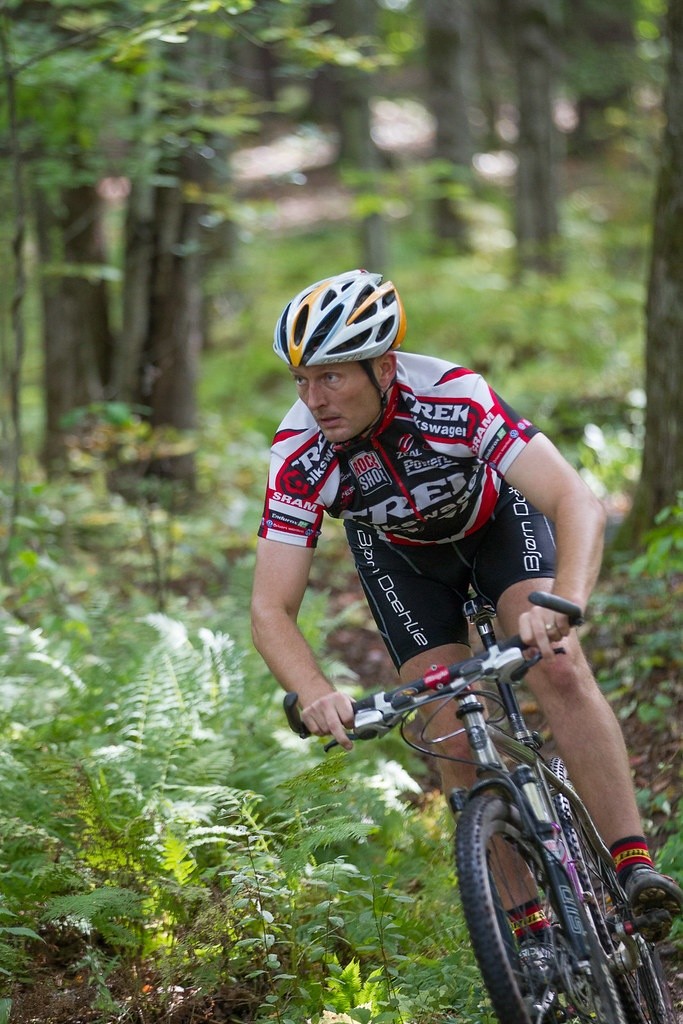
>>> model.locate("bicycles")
[282,590,675,1024]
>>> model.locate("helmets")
[272,269,407,367]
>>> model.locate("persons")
[250,268,683,996]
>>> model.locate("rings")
[545,623,556,629]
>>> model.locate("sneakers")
[622,866,683,943]
[516,936,560,997]
